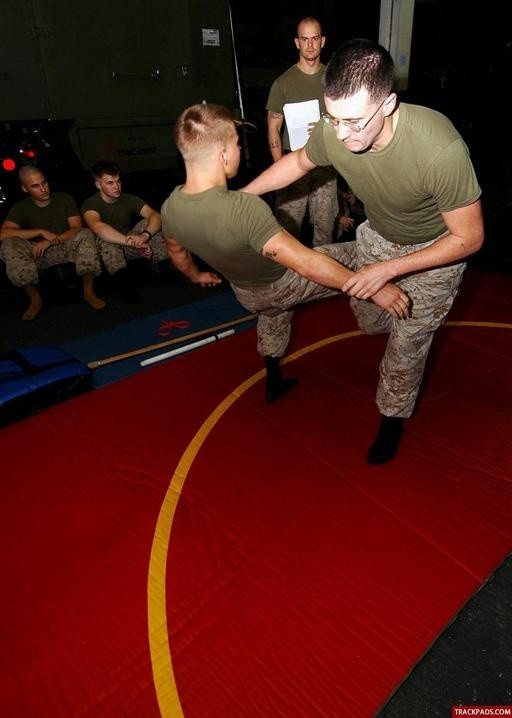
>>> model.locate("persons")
[80,160,177,307]
[231,31,483,467]
[164,94,414,406]
[333,177,363,243]
[262,13,339,254]
[1,162,106,320]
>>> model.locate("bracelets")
[141,229,154,239]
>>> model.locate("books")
[282,99,321,151]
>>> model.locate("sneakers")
[367,414,404,464]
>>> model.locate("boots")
[264,355,298,402]
